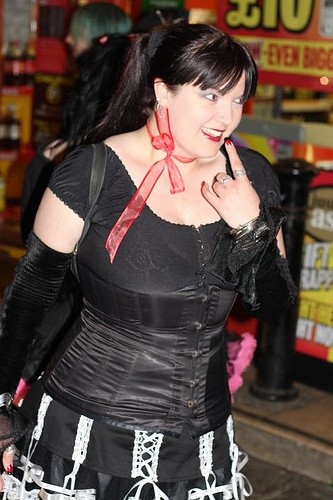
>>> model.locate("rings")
[221,174,234,187]
[233,169,247,178]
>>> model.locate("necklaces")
[106,106,200,263]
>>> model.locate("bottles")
[1,103,22,150]
[22,40,35,84]
[2,37,24,84]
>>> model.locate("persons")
[11,33,156,409]
[65,2,131,59]
[0,23,300,500]
